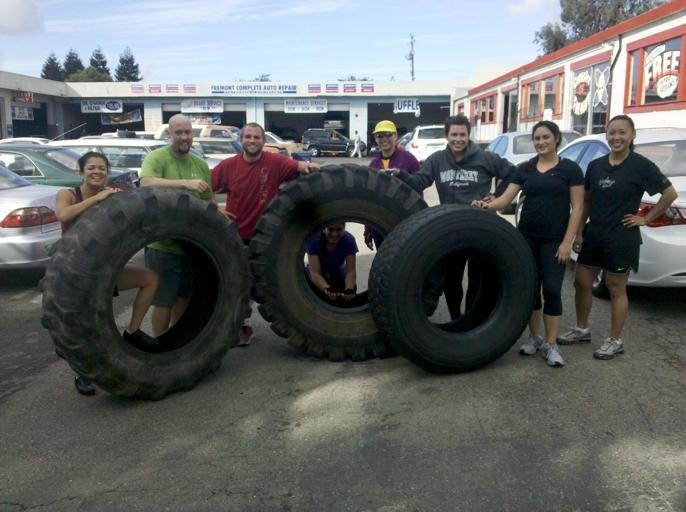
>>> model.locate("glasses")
[376,133,392,137]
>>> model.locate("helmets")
[372,120,396,133]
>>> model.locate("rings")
[632,220,634,223]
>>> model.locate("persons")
[472,120,586,367]
[364,120,422,250]
[210,122,320,348]
[557,116,678,360]
[305,222,358,303]
[139,114,211,336]
[382,113,516,321]
[54,151,159,394]
[351,130,362,159]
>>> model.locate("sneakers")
[124,328,169,352]
[594,336,624,359]
[557,327,591,345]
[74,375,96,394]
[237,326,254,345]
[542,341,564,366]
[520,335,542,355]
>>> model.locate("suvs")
[299,128,367,157]
[405,125,455,163]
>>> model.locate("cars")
[519,127,685,300]
[486,129,584,213]
[0,164,71,269]
[0,123,302,191]
[398,132,414,146]
